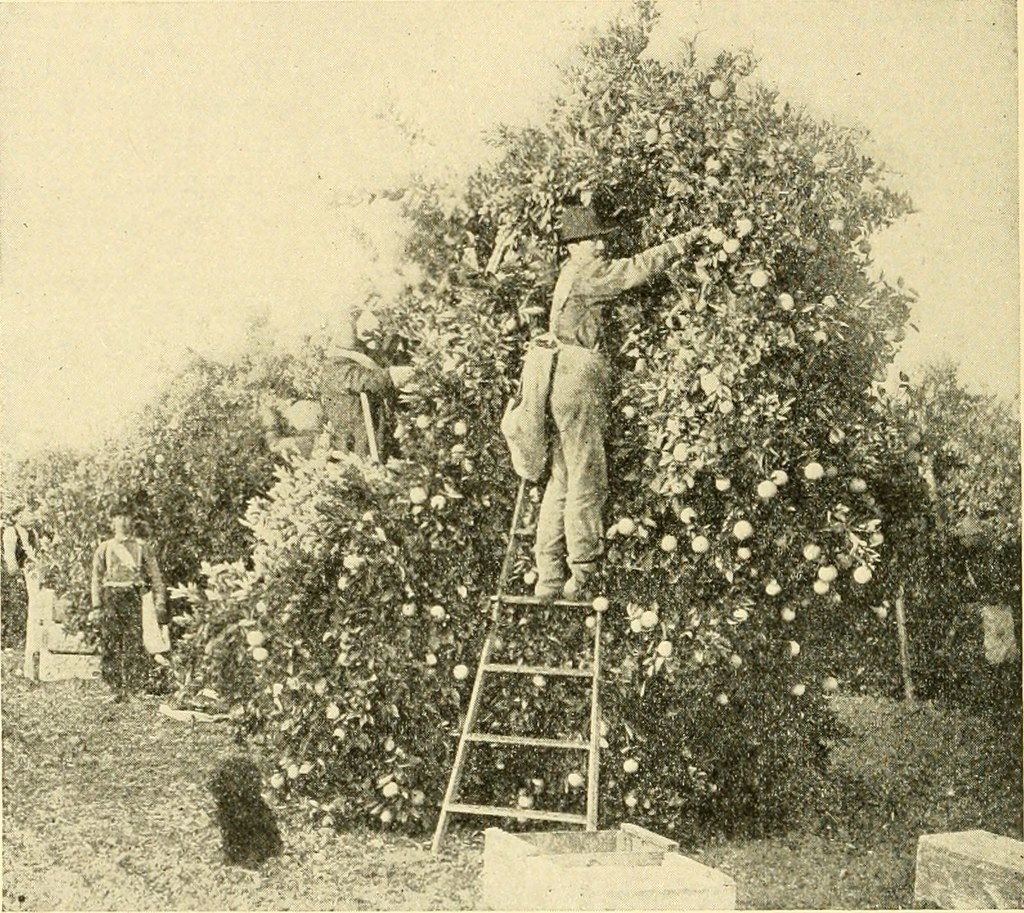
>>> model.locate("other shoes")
[561,574,590,600]
[533,574,569,600]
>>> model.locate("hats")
[554,205,620,244]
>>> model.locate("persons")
[318,323,416,453]
[3,504,38,575]
[91,502,167,703]
[531,205,707,600]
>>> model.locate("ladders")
[428,477,606,858]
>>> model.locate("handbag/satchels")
[142,593,171,654]
